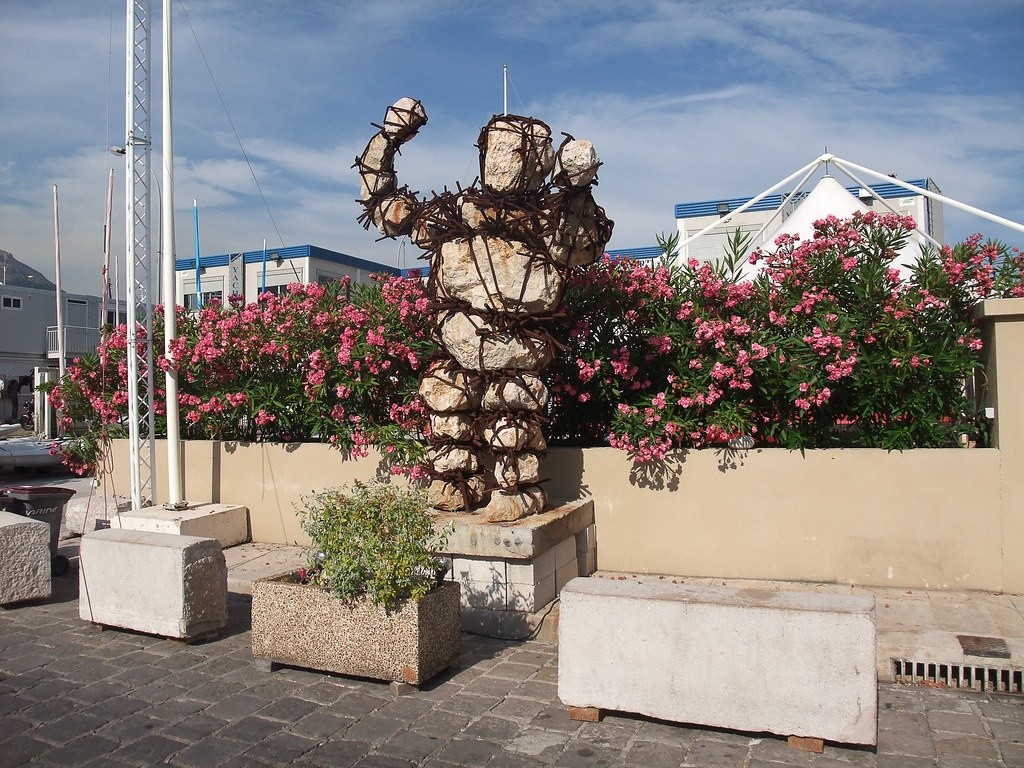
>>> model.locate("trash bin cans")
[0,486,76,576]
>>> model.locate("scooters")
[20,399,34,431]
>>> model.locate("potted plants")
[251,476,462,687]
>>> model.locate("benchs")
[75,529,228,640]
[556,575,879,746]
